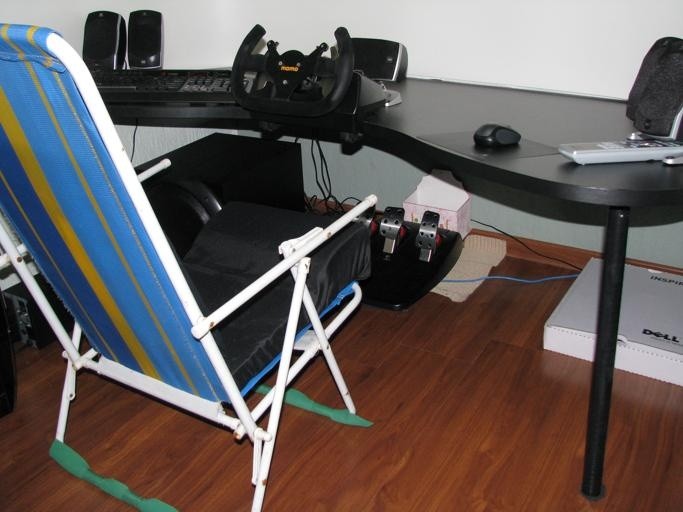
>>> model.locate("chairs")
[0,22,386,512]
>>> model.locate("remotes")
[559,140,683,165]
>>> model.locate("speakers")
[124,10,165,69]
[82,11,126,71]
[628,52,683,140]
[627,36,683,119]
[133,132,304,261]
[330,38,408,83]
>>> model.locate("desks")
[107,66,681,498]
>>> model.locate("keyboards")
[91,69,257,102]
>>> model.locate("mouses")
[473,124,521,145]
